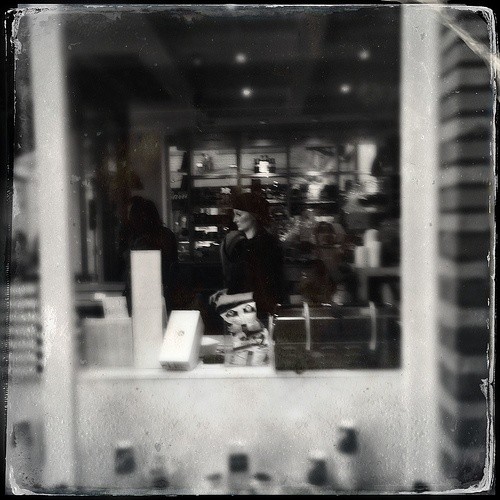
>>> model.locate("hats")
[232,193,270,220]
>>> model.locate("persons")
[231,190,290,313]
[126,197,181,313]
[221,206,248,294]
